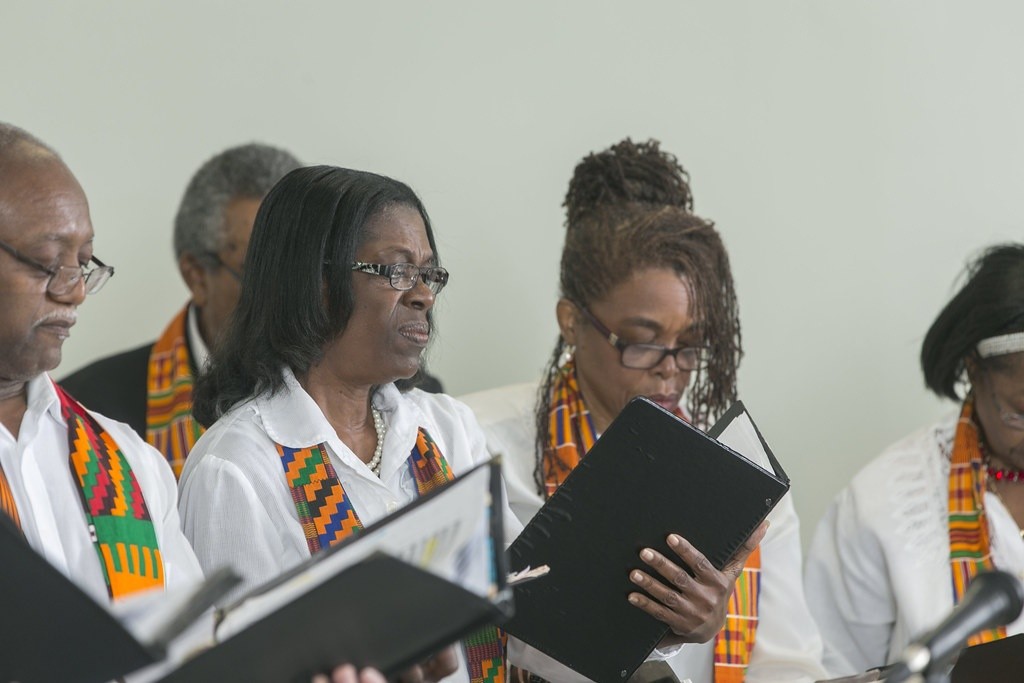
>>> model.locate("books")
[109,452,513,683]
[506,389,793,683]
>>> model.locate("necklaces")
[981,445,1024,493]
[353,405,392,478]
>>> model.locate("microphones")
[886,570,1024,683]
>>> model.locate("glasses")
[1,241,116,297]
[211,254,245,282]
[323,257,450,293]
[564,291,718,373]
[984,369,1024,431]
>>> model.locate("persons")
[1,120,460,683]
[180,164,772,683]
[804,240,1024,683]
[55,138,305,487]
[463,136,825,683]
